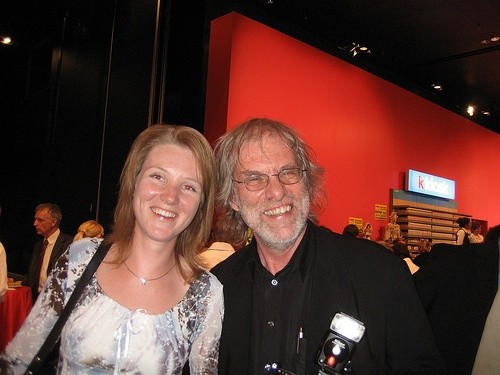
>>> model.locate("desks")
[0,285,33,354]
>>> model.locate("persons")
[0,123,226,375]
[193,210,249,272]
[24,202,73,306]
[455,217,470,245]
[414,225,500,374]
[0,242,9,297]
[468,221,484,244]
[342,224,358,237]
[213,117,438,375]
[392,238,420,291]
[412,238,434,277]
[73,220,104,242]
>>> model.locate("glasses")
[231,168,307,191]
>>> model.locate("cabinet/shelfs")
[390,188,472,258]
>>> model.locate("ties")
[32,240,49,299]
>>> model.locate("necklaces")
[117,242,178,286]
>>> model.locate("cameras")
[315,312,365,375]
